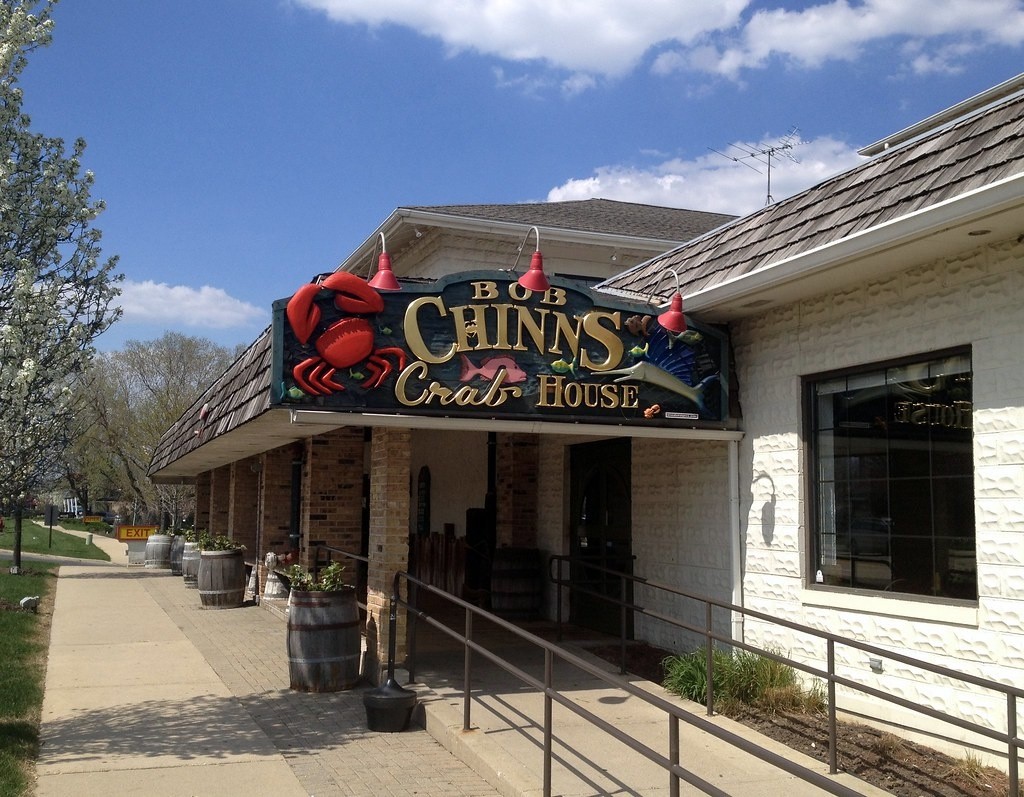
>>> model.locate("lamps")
[512,226,551,292]
[365,231,402,291]
[645,269,688,332]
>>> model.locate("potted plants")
[182,528,211,589]
[191,533,245,610]
[285,559,360,693]
[170,524,194,576]
[144,528,174,569]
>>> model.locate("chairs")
[820,542,978,601]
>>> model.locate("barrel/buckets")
[196,548,245,610]
[143,536,177,570]
[169,536,187,576]
[286,583,360,693]
[182,542,204,588]
